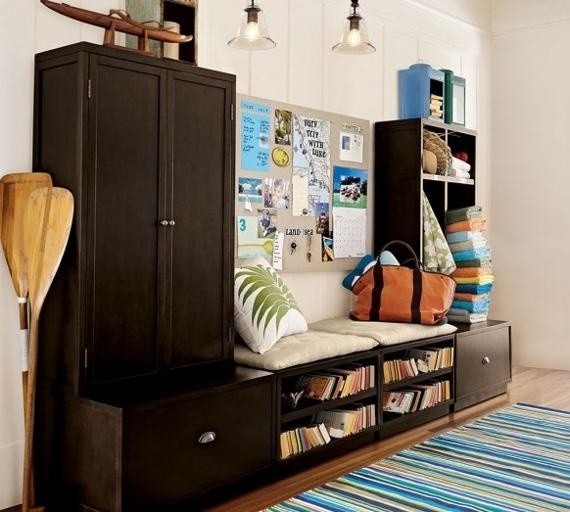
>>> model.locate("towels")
[451,246,491,262]
[451,299,491,313]
[449,236,486,252]
[455,283,492,294]
[447,229,481,243]
[447,205,481,224]
[452,275,495,285]
[446,217,487,233]
[448,308,468,315]
[454,292,489,302]
[447,314,488,323]
[456,257,493,268]
[450,266,495,277]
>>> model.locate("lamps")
[227,2,276,53]
[330,1,376,56]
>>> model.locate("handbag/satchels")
[349,239,456,327]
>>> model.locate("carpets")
[272,400,570,508]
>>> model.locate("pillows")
[228,253,309,354]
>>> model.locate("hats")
[422,128,453,175]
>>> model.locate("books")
[429,93,444,120]
[383,380,450,414]
[280,404,376,460]
[290,364,375,401]
[383,347,459,384]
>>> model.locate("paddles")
[0,173,54,512]
[22,186,75,512]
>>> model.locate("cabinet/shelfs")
[272,331,459,481]
[374,119,514,412]
[31,42,276,507]
